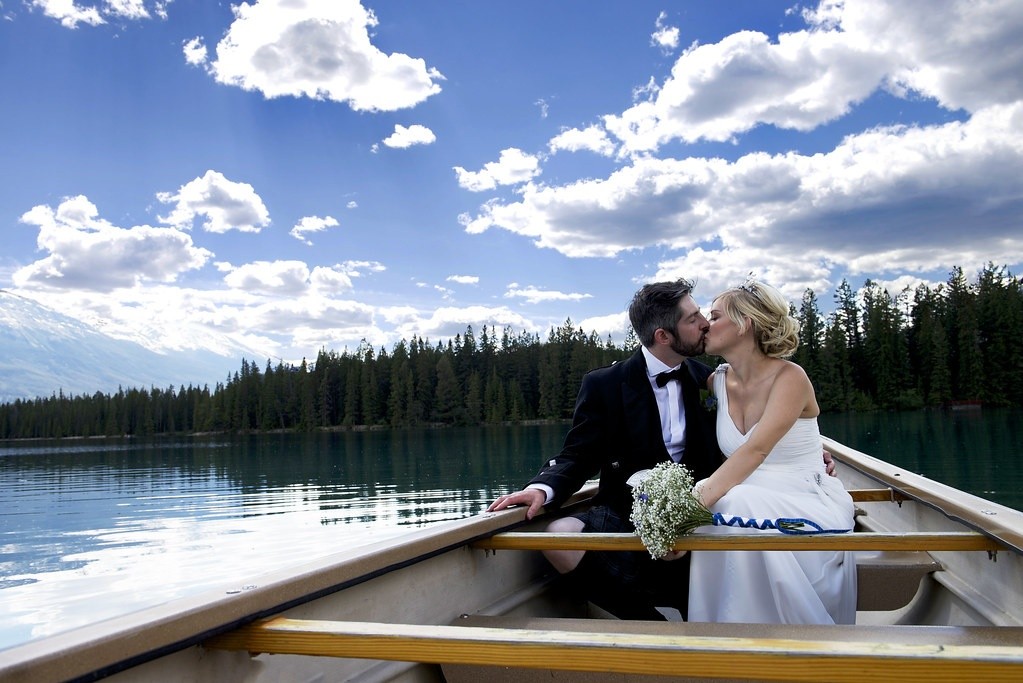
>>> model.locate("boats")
[2,434,1023,683]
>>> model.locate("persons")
[485,278,838,621]
[681,281,856,624]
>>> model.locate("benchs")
[444,617,1023,683]
[854,549,947,612]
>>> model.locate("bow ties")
[656,365,690,388]
